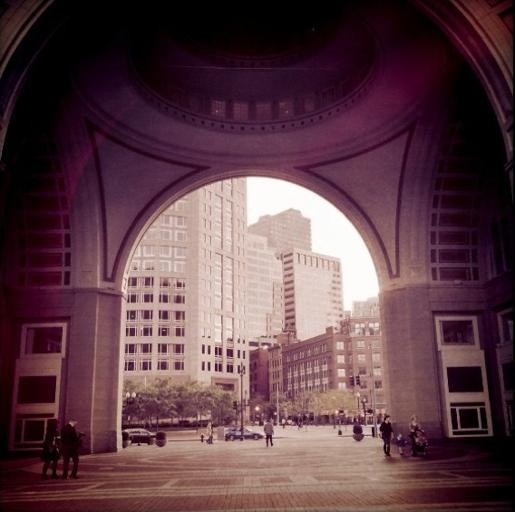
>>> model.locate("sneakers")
[40,473,80,480]
[266,443,274,447]
[385,451,390,456]
[400,450,405,455]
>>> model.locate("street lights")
[126,391,136,427]
[236,360,246,441]
[356,392,361,424]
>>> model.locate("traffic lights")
[349,375,355,386]
[354,374,361,386]
[232,399,237,409]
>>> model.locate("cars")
[123,428,156,445]
[224,424,264,441]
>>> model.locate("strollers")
[411,428,436,457]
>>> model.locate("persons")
[415,433,429,446]
[379,414,395,456]
[408,414,424,457]
[58,416,84,480]
[205,418,214,444]
[38,417,59,479]
[281,417,286,429]
[394,432,409,455]
[263,419,274,447]
